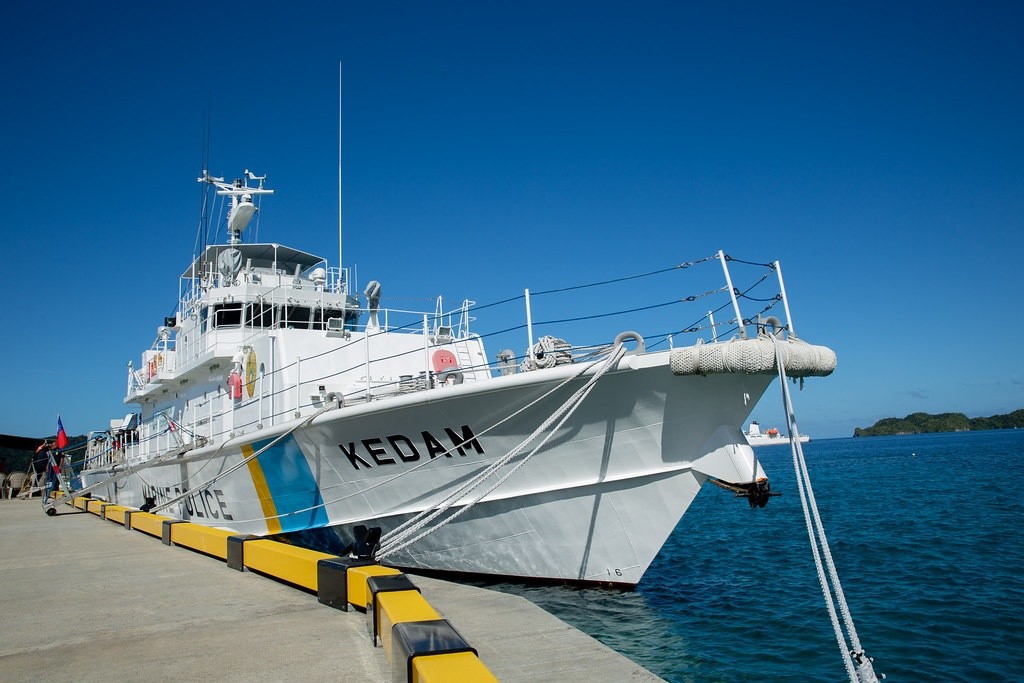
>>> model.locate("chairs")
[0,472,46,499]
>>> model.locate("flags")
[57,414,68,448]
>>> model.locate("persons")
[47,442,65,491]
[94,427,138,463]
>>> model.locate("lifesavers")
[227,375,241,398]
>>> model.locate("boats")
[79,60,838,591]
[741,421,809,446]
